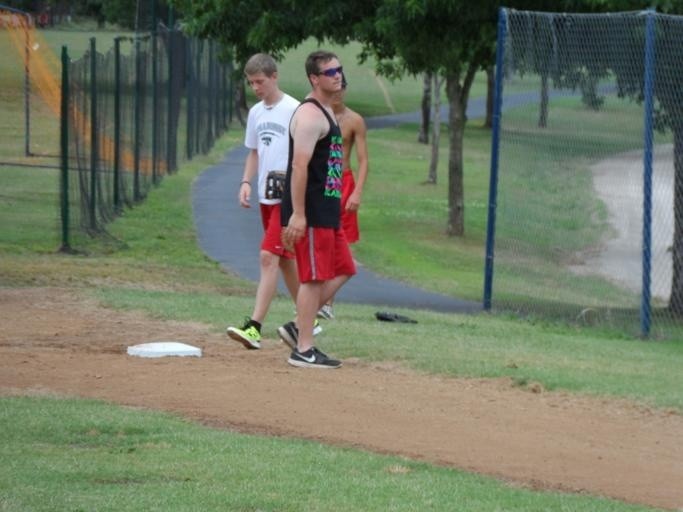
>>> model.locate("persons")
[279,51,358,368]
[225,54,322,351]
[317,74,368,320]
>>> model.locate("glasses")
[319,66,342,76]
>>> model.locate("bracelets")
[240,181,251,185]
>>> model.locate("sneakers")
[287,345,343,369]
[313,303,334,336]
[277,322,298,352]
[227,321,261,349]
